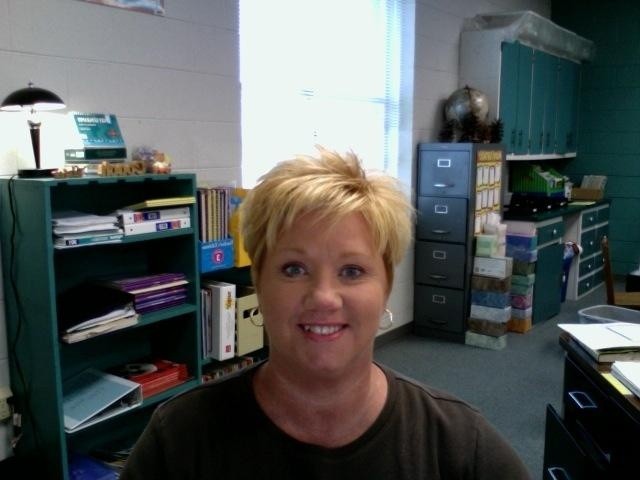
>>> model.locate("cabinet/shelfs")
[414,241,475,289]
[413,284,471,344]
[505,216,563,327]
[542,351,640,480]
[0,173,270,479]
[417,142,506,198]
[555,56,596,159]
[564,202,610,301]
[529,48,560,160]
[416,196,504,244]
[459,41,534,161]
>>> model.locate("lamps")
[0,81,66,178]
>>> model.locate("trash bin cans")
[577,305,640,323]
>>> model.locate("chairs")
[603,235,640,310]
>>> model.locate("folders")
[118,207,191,237]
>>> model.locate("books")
[69,436,136,480]
[198,184,236,369]
[60,261,189,343]
[556,322,640,403]
[51,197,197,247]
[113,354,194,396]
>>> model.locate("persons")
[119,140,534,480]
